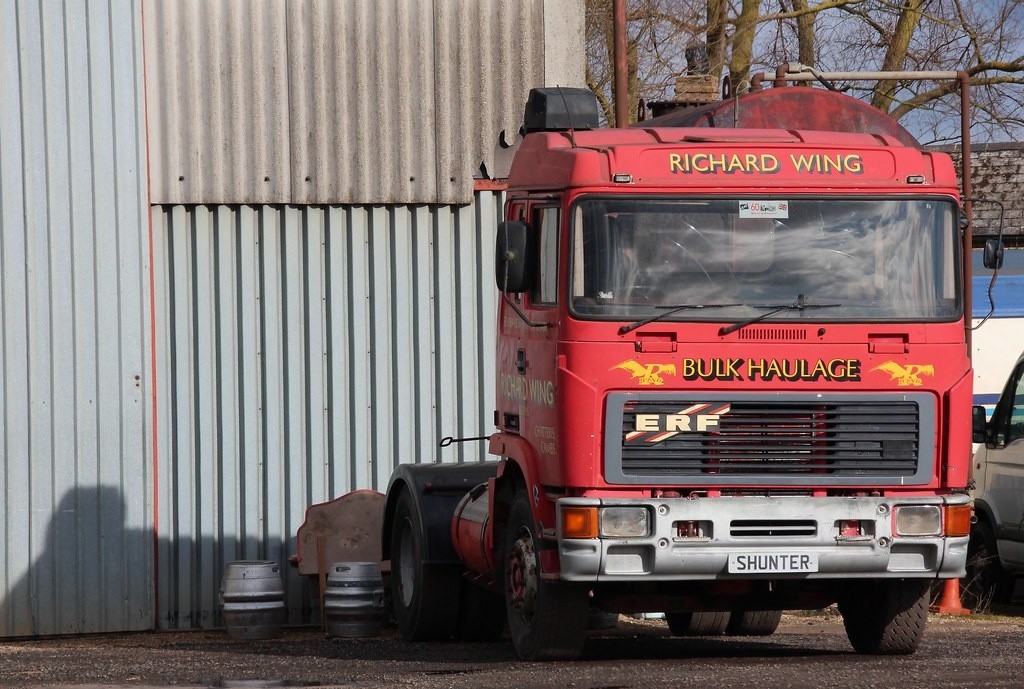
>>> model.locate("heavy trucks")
[377,83,977,658]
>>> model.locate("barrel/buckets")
[323,563,390,637]
[212,560,298,643]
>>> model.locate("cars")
[972,351,1024,607]
